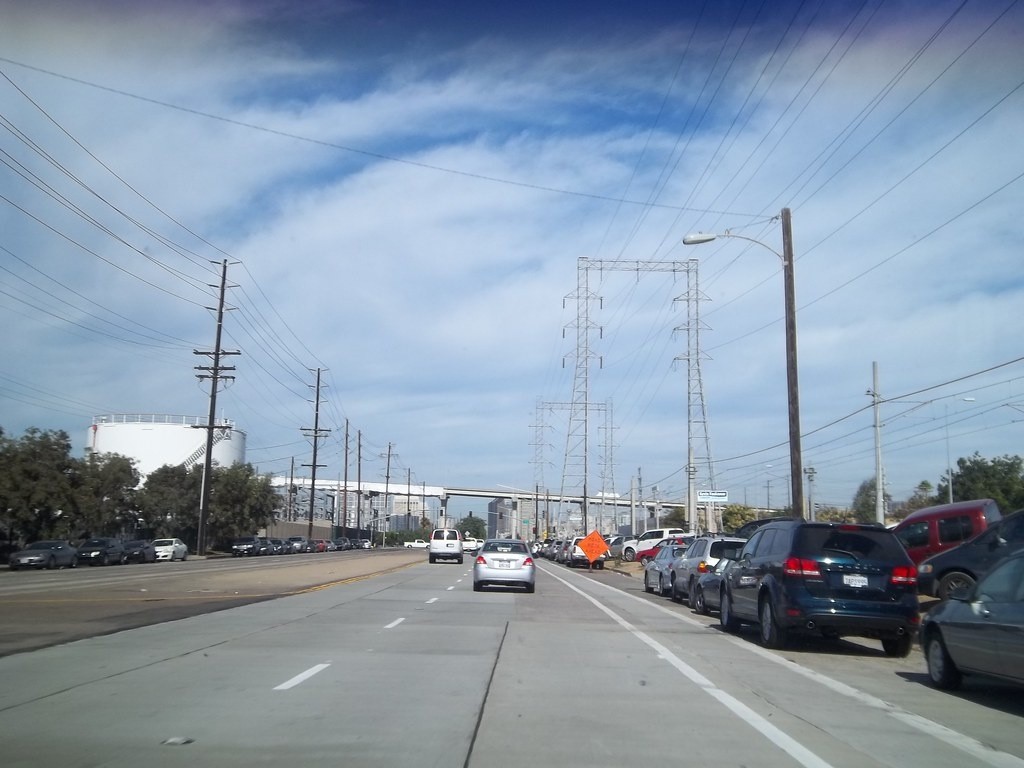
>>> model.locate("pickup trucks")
[404,538,431,549]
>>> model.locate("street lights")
[683,230,803,519]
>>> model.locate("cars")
[150,538,189,563]
[636,535,695,567]
[693,547,742,616]
[8,538,80,570]
[73,536,126,567]
[475,538,484,551]
[531,534,639,570]
[644,543,690,598]
[460,537,479,552]
[470,539,540,592]
[230,532,373,557]
[122,538,156,565]
[916,545,1024,692]
[914,507,1024,598]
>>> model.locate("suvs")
[621,527,686,563]
[719,517,921,657]
[670,532,749,605]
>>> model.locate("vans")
[429,528,466,564]
[887,499,1002,572]
